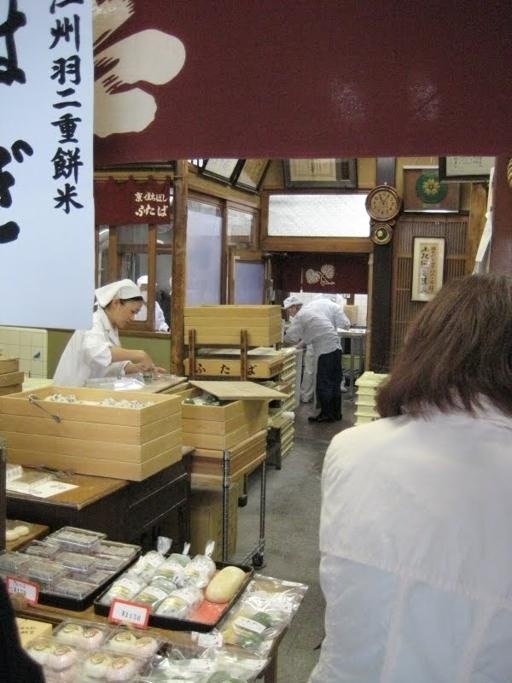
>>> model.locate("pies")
[5,526,30,540]
[25,551,281,681]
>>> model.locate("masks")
[140,291,148,304]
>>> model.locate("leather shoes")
[308,413,335,423]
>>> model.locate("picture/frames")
[411,235,446,301]
[398,153,494,214]
[199,158,271,193]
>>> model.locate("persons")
[128,273,169,332]
[52,278,166,390]
[292,293,352,405]
[300,269,512,683]
[280,295,343,423]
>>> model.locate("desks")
[1,442,308,683]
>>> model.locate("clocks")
[365,185,403,222]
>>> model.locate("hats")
[95,279,143,309]
[283,296,303,310]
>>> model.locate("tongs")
[25,392,64,425]
[33,463,76,479]
